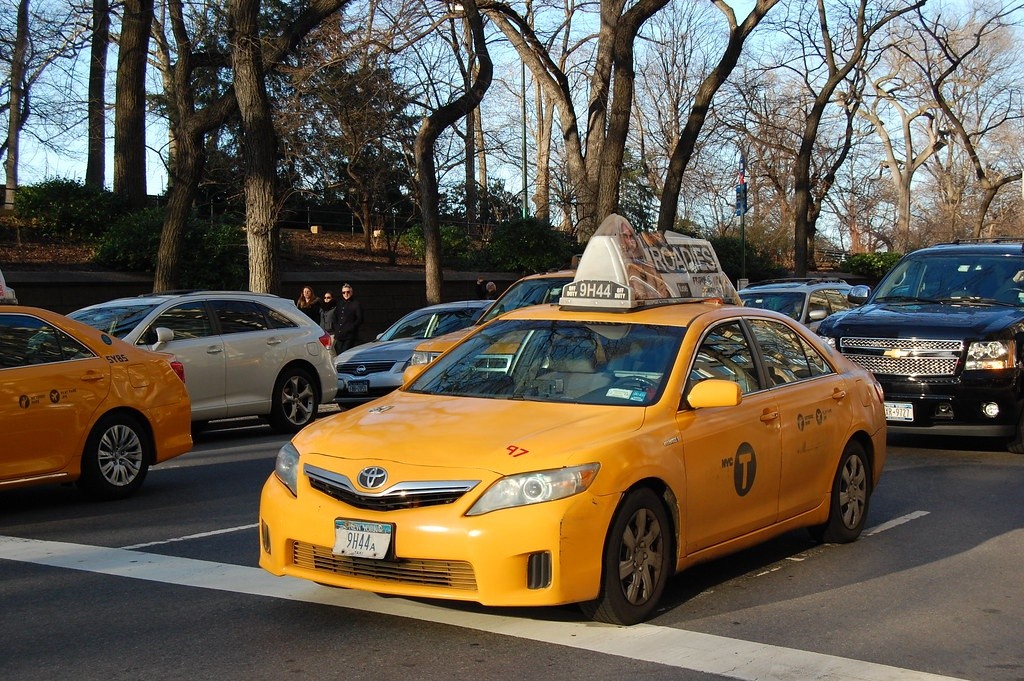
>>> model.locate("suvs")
[816,237,1024,453]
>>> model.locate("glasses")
[324,297,330,299]
[342,291,350,294]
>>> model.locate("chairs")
[536,336,616,399]
[607,340,665,372]
[934,267,966,298]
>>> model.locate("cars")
[0,267,195,501]
[733,278,860,361]
[20,288,339,432]
[331,298,495,411]
[256,211,889,625]
[401,253,628,388]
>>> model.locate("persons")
[993,263,1024,306]
[787,296,804,320]
[470,274,502,300]
[295,283,363,361]
